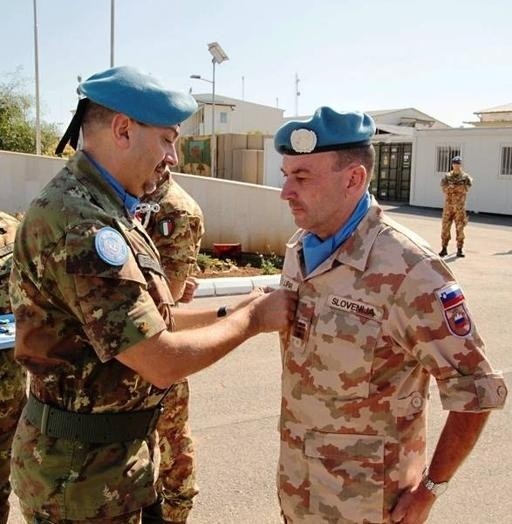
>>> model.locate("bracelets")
[216,302,231,322]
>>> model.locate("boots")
[457,247,466,257]
[439,246,448,257]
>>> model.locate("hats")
[451,156,462,164]
[78,66,198,124]
[274,106,378,156]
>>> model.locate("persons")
[7,64,303,524]
[131,162,207,524]
[272,105,509,524]
[0,208,39,524]
[440,156,475,258]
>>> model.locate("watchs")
[419,469,450,498]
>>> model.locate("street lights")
[190,74,217,178]
